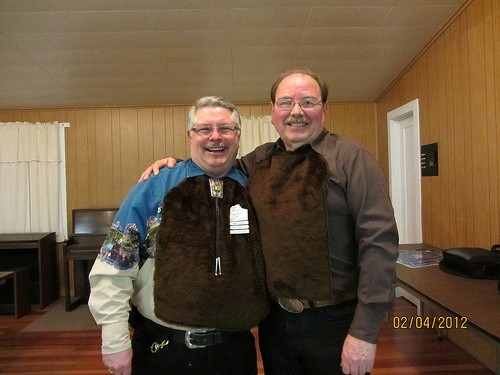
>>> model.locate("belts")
[141,317,249,349]
[273,289,358,313]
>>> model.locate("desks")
[387,242,500,375]
[56,240,103,312]
[0,232,58,309]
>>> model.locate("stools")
[0,266,18,318]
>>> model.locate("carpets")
[21,296,102,330]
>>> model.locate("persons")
[88,96,272,375]
[137,66,399,375]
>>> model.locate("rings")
[106,368,115,375]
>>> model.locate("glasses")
[190,125,241,136]
[274,99,322,110]
